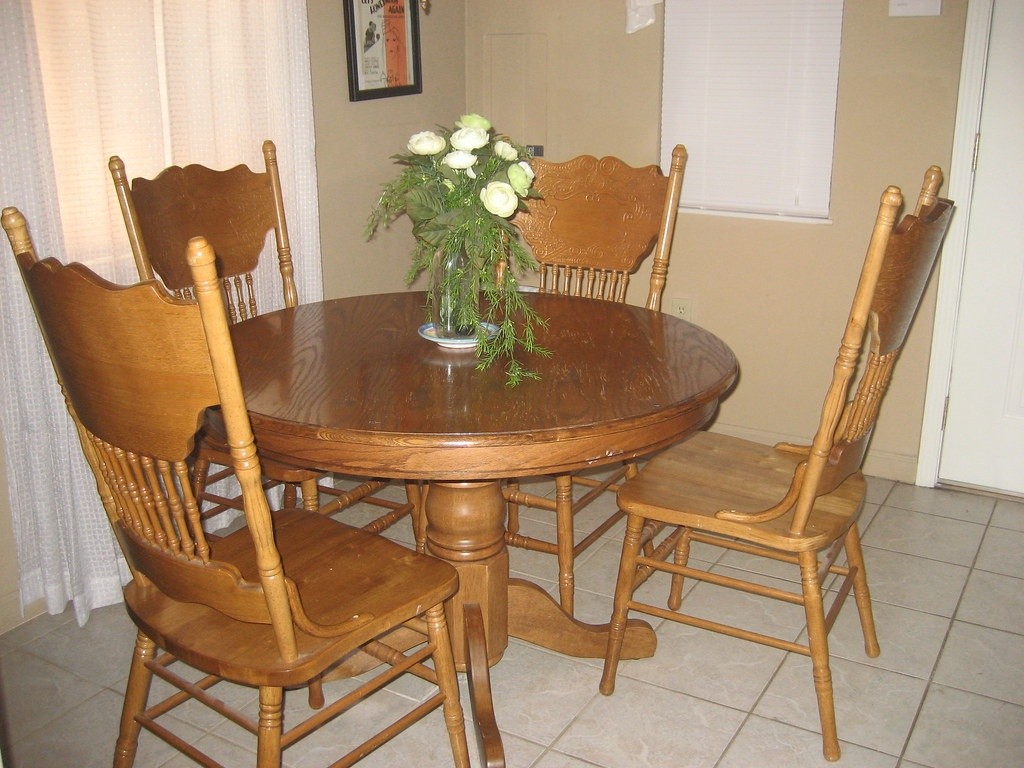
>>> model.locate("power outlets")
[526,146,544,157]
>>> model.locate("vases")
[430,231,481,338]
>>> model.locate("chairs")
[415,143,688,617]
[598,164,957,761]
[1,206,473,768]
[107,139,423,708]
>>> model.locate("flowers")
[363,113,549,387]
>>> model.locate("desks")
[198,291,741,691]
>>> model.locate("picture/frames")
[343,0,423,102]
